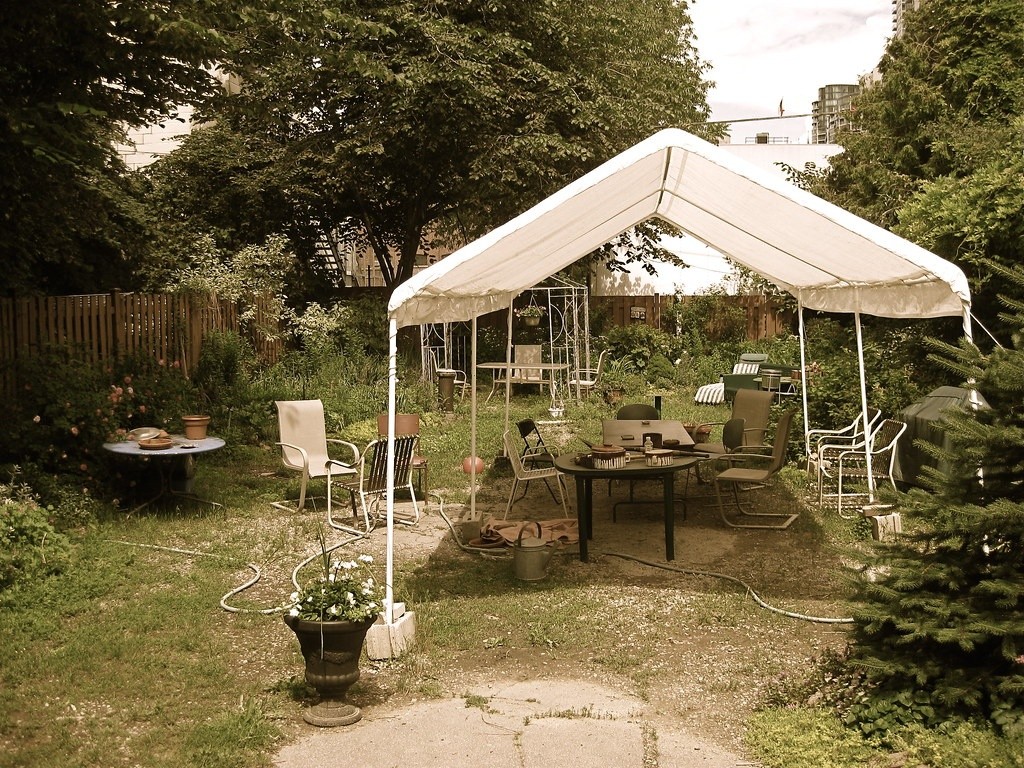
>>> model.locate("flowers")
[514,299,549,321]
[279,495,381,622]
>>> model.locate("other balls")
[463,457,483,474]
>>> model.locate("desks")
[753,378,796,407]
[105,431,227,516]
[602,420,695,523]
[556,450,697,560]
[476,361,572,409]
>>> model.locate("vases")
[525,315,542,327]
[283,616,377,726]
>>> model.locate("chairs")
[694,352,769,404]
[696,388,801,529]
[269,398,430,538]
[502,421,572,523]
[806,407,908,518]
[511,345,543,396]
[566,348,608,405]
[428,350,471,405]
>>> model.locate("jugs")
[514,521,562,581]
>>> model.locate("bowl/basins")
[645,450,674,466]
[592,442,631,469]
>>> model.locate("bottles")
[644,437,653,452]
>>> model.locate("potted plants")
[184,384,211,438]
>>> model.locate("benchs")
[723,363,800,406]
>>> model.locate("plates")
[138,439,173,449]
